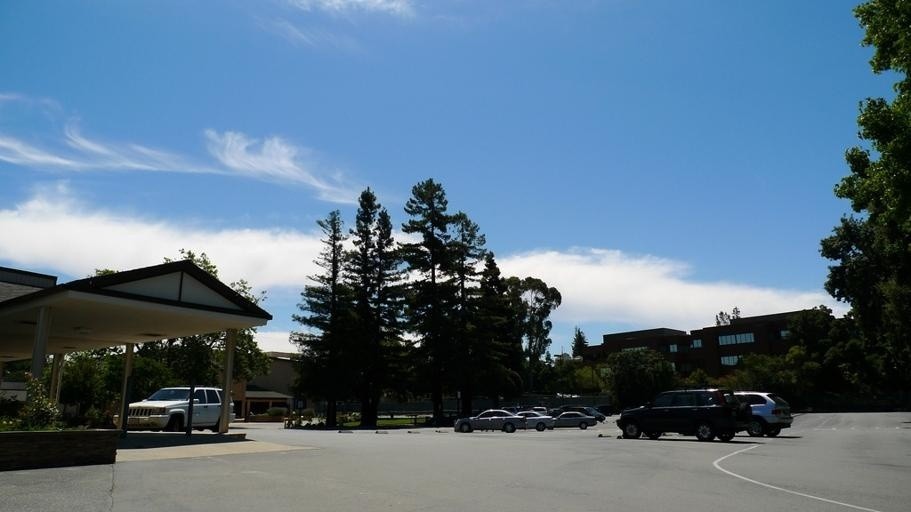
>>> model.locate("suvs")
[111,382,237,433]
[615,386,755,443]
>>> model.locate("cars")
[551,410,598,431]
[448,408,528,434]
[510,410,553,432]
[501,405,607,424]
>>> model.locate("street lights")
[554,353,564,406]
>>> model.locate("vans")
[727,390,794,438]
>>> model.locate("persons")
[286,411,312,428]
